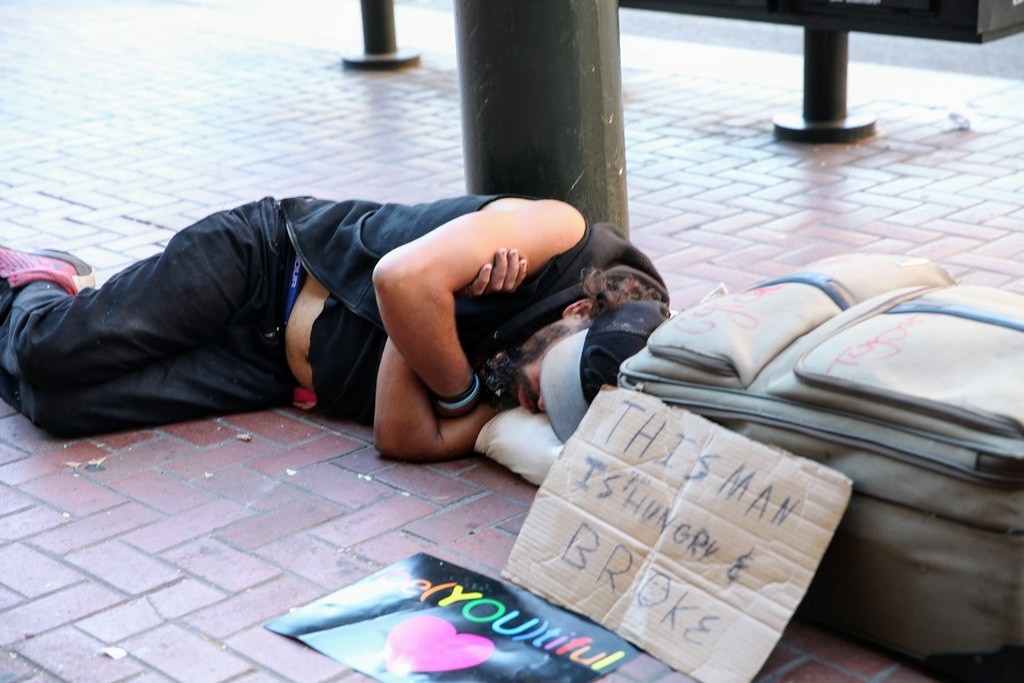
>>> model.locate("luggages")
[615,254,1024,683]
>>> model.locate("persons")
[0,193,671,465]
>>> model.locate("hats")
[540,301,668,444]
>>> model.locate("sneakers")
[0,244,95,297]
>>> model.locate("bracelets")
[434,373,479,410]
